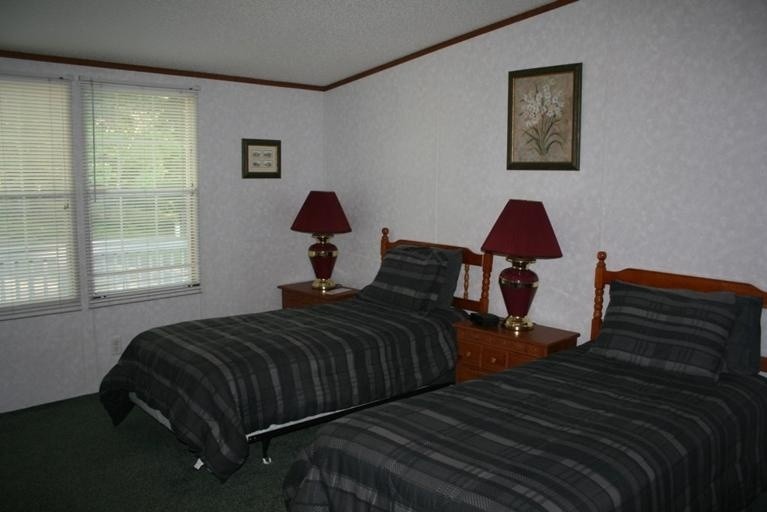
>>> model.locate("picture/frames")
[505,62,584,172]
[240,137,283,181]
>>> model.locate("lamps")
[289,189,353,290]
[479,196,564,331]
